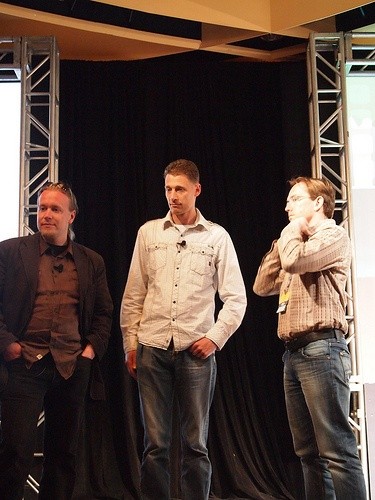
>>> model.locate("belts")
[286,331,343,349]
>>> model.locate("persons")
[253,176,367,500]
[118,159,249,499]
[0,181,115,500]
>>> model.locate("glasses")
[43,180,70,191]
[286,196,314,206]
